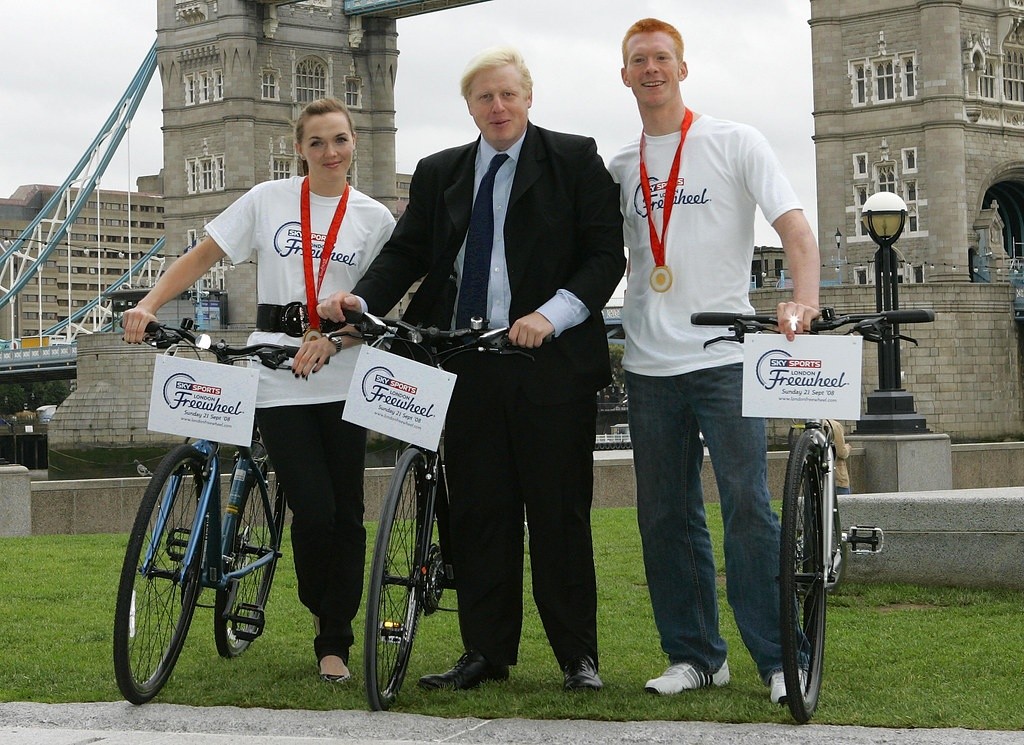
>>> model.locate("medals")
[302,330,321,343]
[650,266,673,294]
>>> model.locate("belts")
[256,301,349,338]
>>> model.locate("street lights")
[847,191,933,435]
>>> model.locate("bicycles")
[690,306,938,726]
[112,316,331,706]
[343,305,554,711]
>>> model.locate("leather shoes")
[561,654,603,691]
[416,647,511,692]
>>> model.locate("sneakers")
[646,658,730,697]
[769,669,807,706]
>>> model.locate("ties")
[451,153,509,359]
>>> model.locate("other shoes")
[318,657,350,682]
[312,613,321,638]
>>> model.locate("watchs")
[323,331,342,354]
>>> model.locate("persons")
[609,18,819,710]
[819,419,852,496]
[316,50,628,696]
[123,97,397,683]
